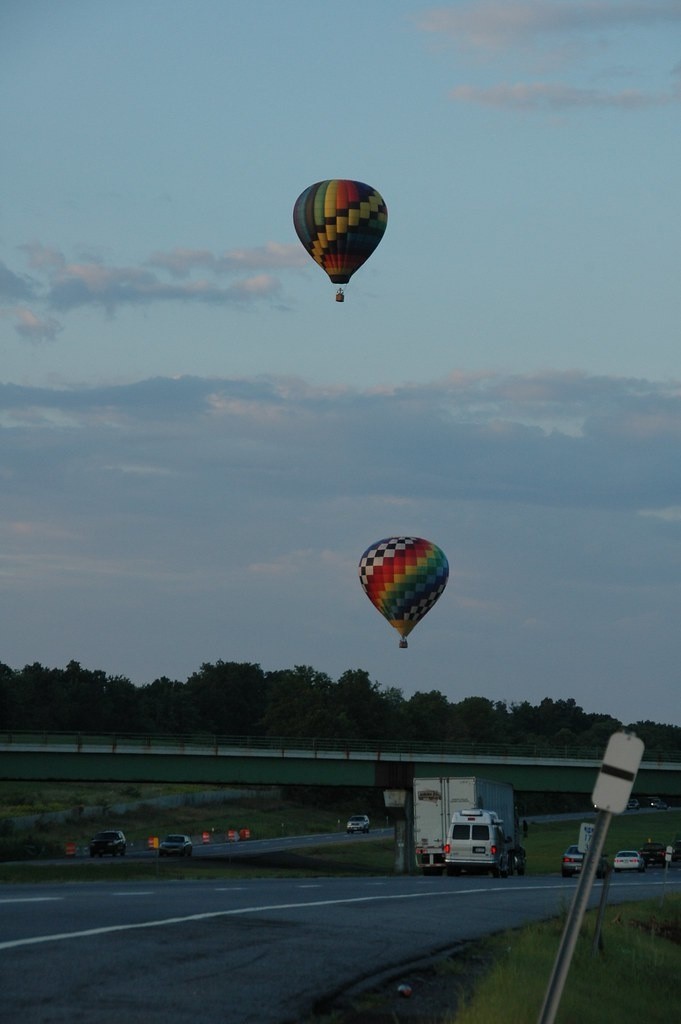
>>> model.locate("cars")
[672,840,681,861]
[614,849,646,874]
[515,846,527,876]
[158,834,193,858]
[628,796,668,811]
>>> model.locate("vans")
[444,808,507,878]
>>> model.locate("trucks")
[411,776,518,875]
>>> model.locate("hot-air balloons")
[358,535,451,651]
[291,179,389,306]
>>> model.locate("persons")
[522,820,528,837]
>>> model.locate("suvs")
[637,842,671,868]
[89,831,127,859]
[346,814,370,835]
[561,843,610,878]
